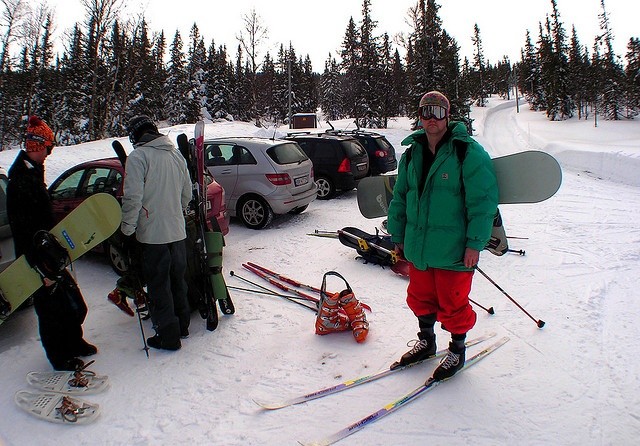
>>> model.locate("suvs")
[279,131,369,199]
[179,137,318,228]
[323,129,397,175]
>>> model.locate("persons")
[384,87,500,381]
[107,271,152,318]
[6,112,98,374]
[113,111,194,351]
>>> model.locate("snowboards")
[357,151,562,219]
[0,193,122,326]
[339,227,394,266]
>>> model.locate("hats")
[419,91,450,110]
[24,116,54,152]
[127,115,161,144]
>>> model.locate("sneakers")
[400,333,436,366]
[432,341,466,379]
[108,287,134,317]
[147,333,182,351]
[70,339,97,356]
[53,356,83,371]
[134,288,147,307]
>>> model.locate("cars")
[49,159,230,276]
[0,168,34,309]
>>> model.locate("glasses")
[27,135,53,156]
[421,106,447,120]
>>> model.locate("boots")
[339,290,369,343]
[315,291,353,335]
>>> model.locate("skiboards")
[242,262,372,316]
[253,333,510,446]
[177,121,235,331]
[112,140,152,321]
